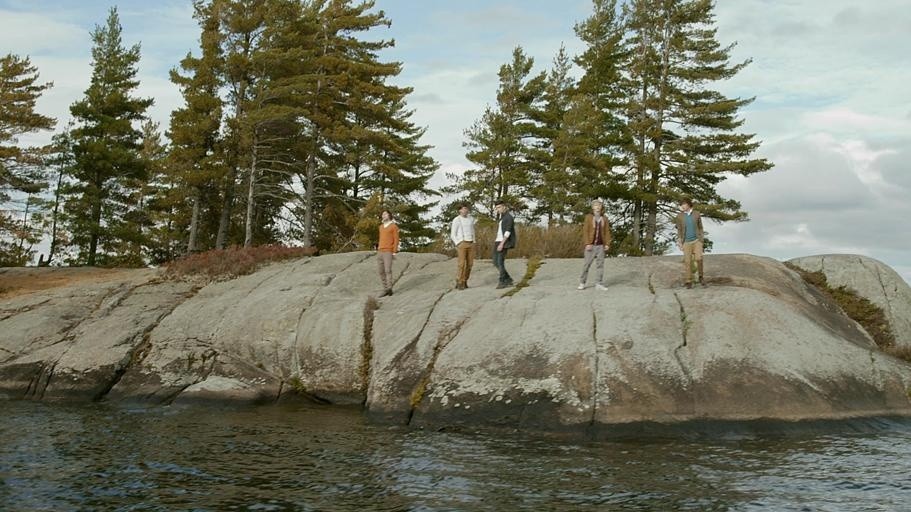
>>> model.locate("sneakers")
[388,289,392,296]
[687,283,691,289]
[457,280,464,290]
[699,275,704,286]
[496,282,504,288]
[596,283,607,290]
[577,284,584,289]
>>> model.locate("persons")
[578,198,613,291]
[491,200,516,289]
[450,202,476,291]
[376,209,400,298]
[676,197,707,290]
